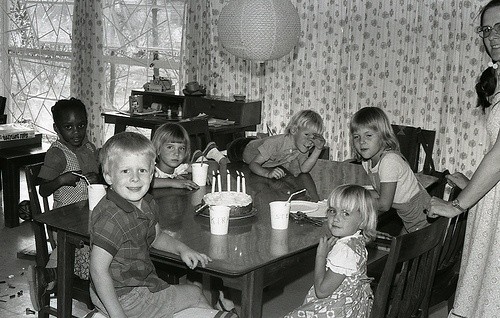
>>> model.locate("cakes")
[200,191,253,217]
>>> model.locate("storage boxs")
[0,131,43,150]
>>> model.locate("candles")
[227,169,230,192]
[241,172,246,193]
[217,170,222,192]
[236,170,240,192]
[212,171,215,193]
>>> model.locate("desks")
[35,158,439,318]
[0,142,52,228]
[102,110,256,151]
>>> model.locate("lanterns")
[217,0,301,61]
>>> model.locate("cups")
[191,163,209,187]
[191,187,206,206]
[151,102,162,110]
[129,95,143,114]
[87,184,108,211]
[269,201,290,230]
[209,206,231,235]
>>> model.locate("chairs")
[183,118,210,151]
[391,125,436,175]
[367,186,469,318]
[24,163,94,318]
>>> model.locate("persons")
[151,123,191,179]
[192,109,326,179]
[88,132,215,318]
[350,107,432,234]
[27,97,99,312]
[425,0,500,318]
[284,183,378,318]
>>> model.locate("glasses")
[477,22,500,38]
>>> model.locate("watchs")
[451,199,467,213]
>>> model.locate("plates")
[306,203,327,217]
[290,200,319,213]
[193,204,260,220]
[134,110,163,115]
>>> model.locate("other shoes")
[28,264,56,311]
[191,142,216,163]
